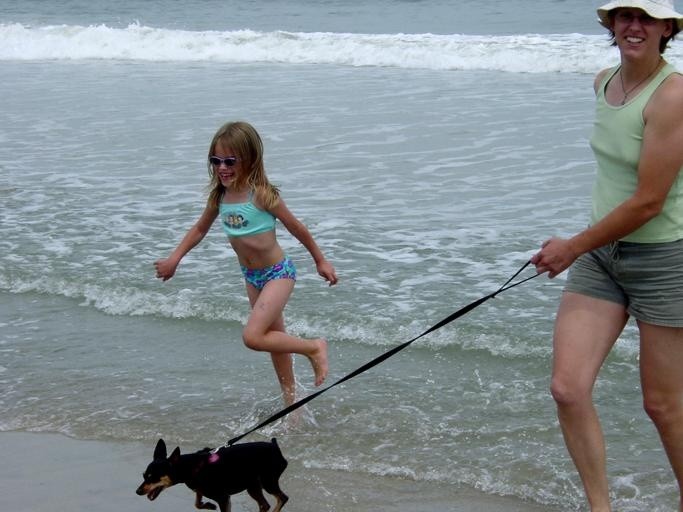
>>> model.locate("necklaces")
[619,59,662,104]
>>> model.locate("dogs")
[135,438,289,512]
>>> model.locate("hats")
[596,0,683,33]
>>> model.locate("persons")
[152,123,340,406]
[530,0,683,512]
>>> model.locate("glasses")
[210,153,251,167]
[614,11,659,26]
